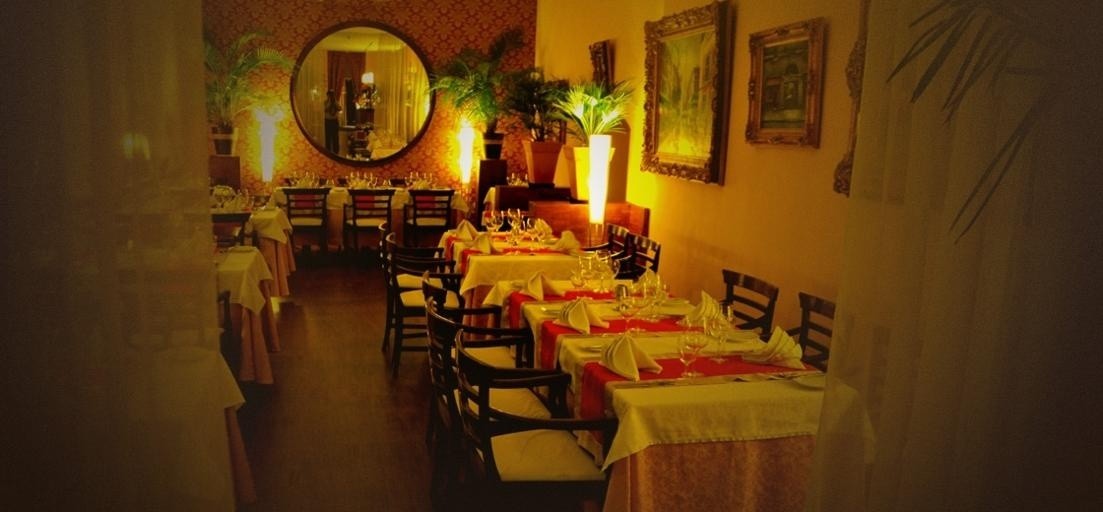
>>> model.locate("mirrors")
[293,16,440,170]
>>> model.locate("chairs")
[283,174,456,273]
[580,219,659,284]
[720,268,834,373]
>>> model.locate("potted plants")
[416,23,639,202]
[202,21,298,156]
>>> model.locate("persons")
[324,89,343,155]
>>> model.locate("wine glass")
[344,169,379,189]
[484,207,548,257]
[402,171,438,191]
[286,169,335,189]
[505,171,528,186]
[571,248,733,382]
[212,187,255,215]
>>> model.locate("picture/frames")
[641,0,739,189]
[743,13,828,150]
